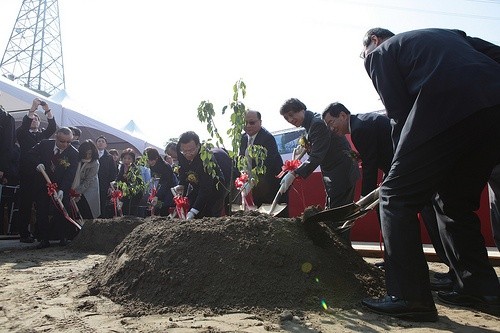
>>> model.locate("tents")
[62,101,165,161]
[1,74,62,130]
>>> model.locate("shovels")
[303,185,380,226]
[256,145,307,217]
[14,142,83,230]
[67,186,90,227]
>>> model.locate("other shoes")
[21,233,52,248]
[429,275,452,291]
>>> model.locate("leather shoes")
[435,290,500,316]
[361,297,438,322]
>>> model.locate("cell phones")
[38,100,45,106]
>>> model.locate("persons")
[143,147,178,217]
[207,147,232,217]
[108,148,118,170]
[68,126,82,153]
[15,96,57,243]
[135,155,151,210]
[279,98,360,246]
[173,130,226,220]
[358,28,500,324]
[0,104,16,183]
[69,138,100,233]
[321,101,457,289]
[96,135,117,219]
[166,143,193,197]
[233,109,290,220]
[164,153,174,171]
[111,148,145,218]
[25,126,80,250]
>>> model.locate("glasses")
[180,148,198,155]
[246,120,260,124]
[57,140,72,143]
[359,45,368,59]
